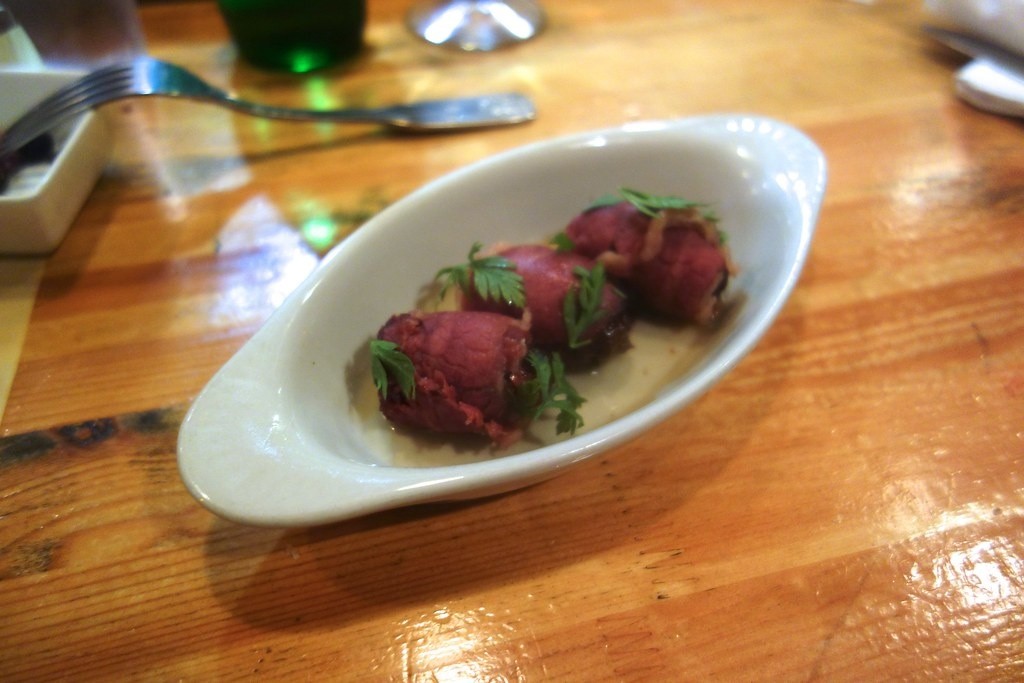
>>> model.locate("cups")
[2,0,146,72]
[211,0,366,75]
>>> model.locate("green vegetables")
[368,184,727,437]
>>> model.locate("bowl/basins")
[175,113,828,527]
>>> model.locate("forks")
[0,56,536,158]
